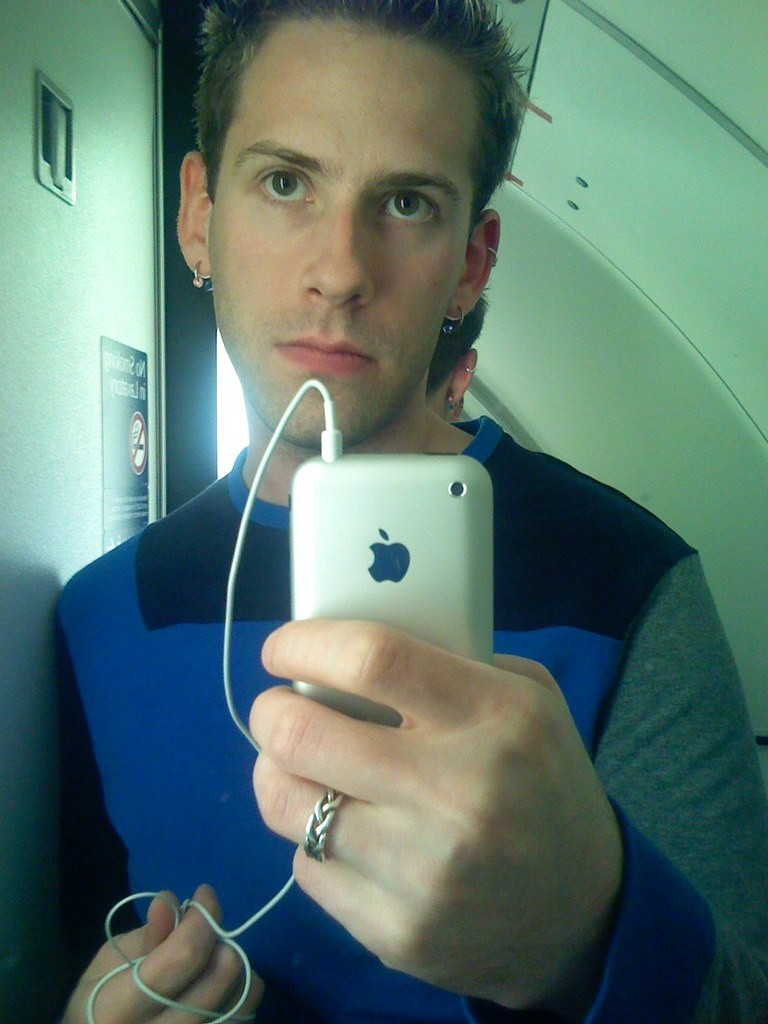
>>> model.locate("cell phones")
[292,453,495,724]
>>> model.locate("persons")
[424,293,490,423]
[49,0,768,1024]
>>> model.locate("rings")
[303,788,345,862]
